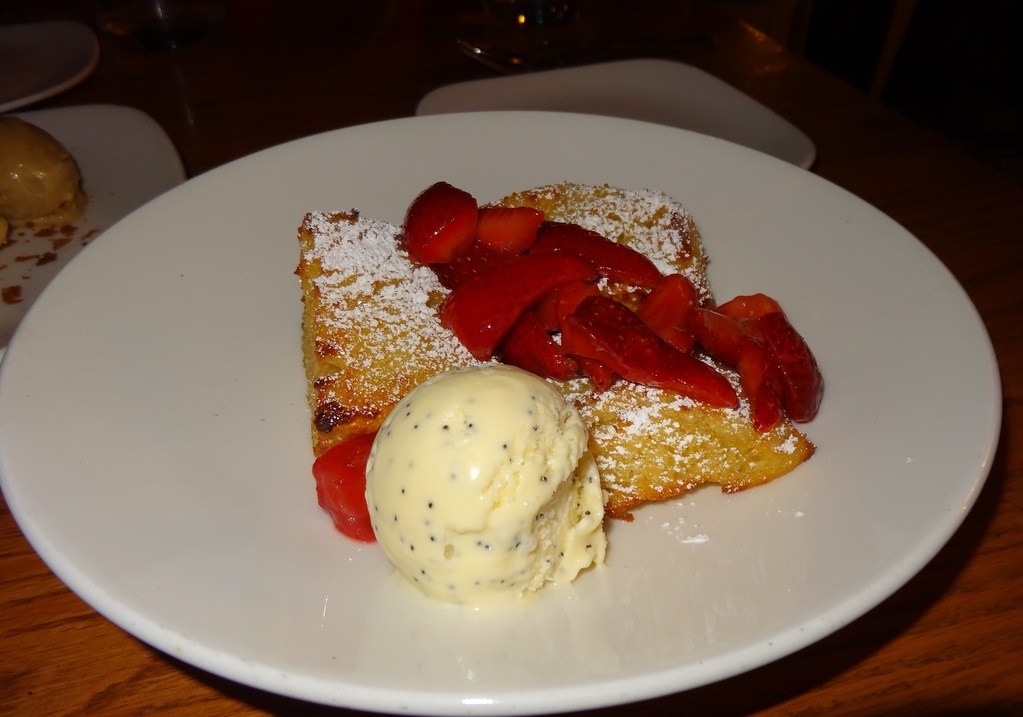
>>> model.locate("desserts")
[292,184,824,606]
[0,117,87,244]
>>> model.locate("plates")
[417,59,815,169]
[0,20,98,110]
[0,110,1003,716]
[0,104,186,349]
